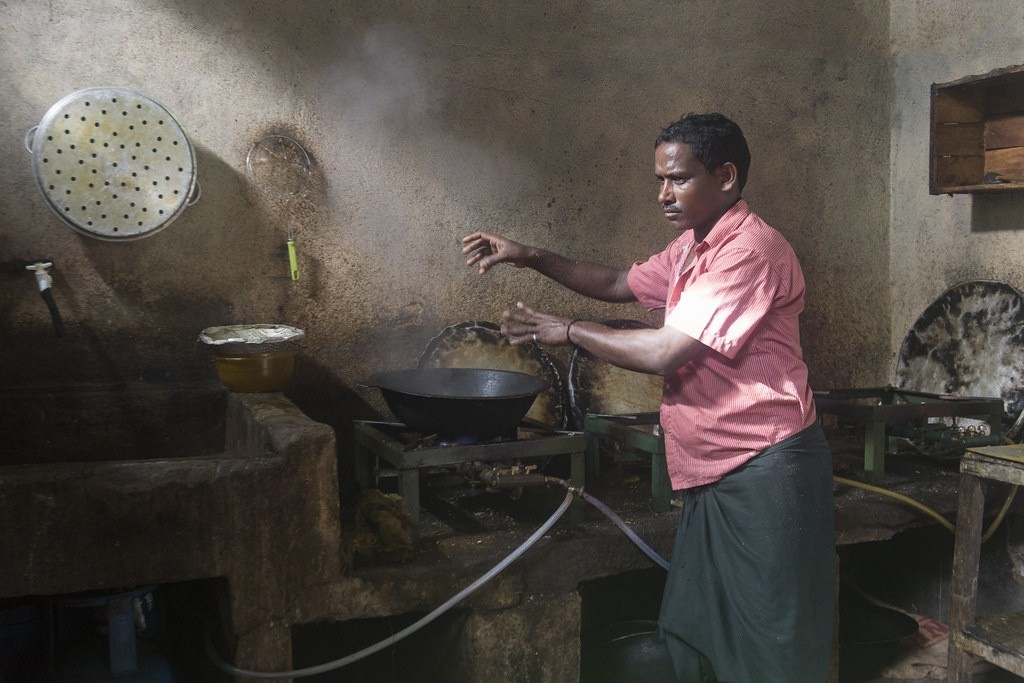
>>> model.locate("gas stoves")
[353,417,587,467]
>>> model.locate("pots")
[368,368,551,442]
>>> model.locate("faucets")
[24,262,54,291]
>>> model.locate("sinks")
[0,379,343,601]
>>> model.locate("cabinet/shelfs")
[946,443,1024,682]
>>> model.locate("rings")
[533,334,537,342]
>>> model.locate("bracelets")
[567,319,582,345]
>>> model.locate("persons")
[463,112,839,682]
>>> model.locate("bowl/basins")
[199,323,305,354]
[211,351,300,393]
